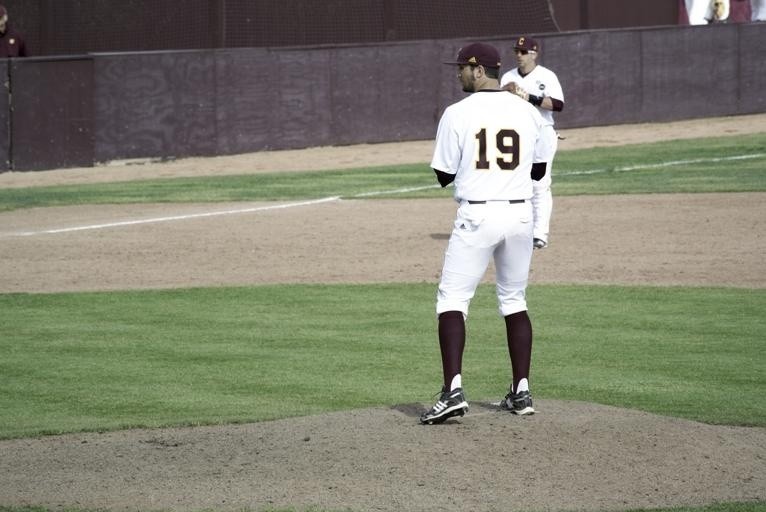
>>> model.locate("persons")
[0,6,27,56]
[499,37,565,250]
[420,43,549,425]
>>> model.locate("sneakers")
[533,238,546,248]
[500,390,535,415]
[420,387,469,424]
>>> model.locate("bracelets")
[529,94,544,107]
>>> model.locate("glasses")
[515,49,535,55]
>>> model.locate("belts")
[469,200,524,204]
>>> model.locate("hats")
[514,37,539,53]
[443,43,501,69]
